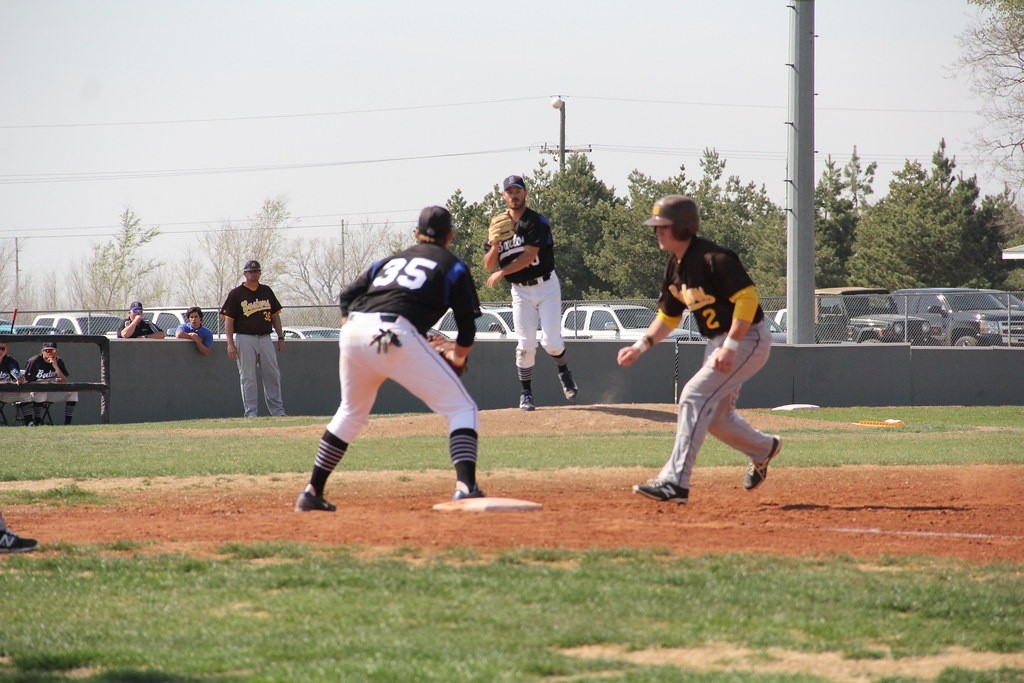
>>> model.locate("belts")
[255,333,268,337]
[381,315,397,323]
[514,274,550,286]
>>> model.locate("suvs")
[891,287,1024,349]
[815,287,937,346]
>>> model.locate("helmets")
[642,195,699,241]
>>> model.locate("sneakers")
[558,369,578,400]
[0,530,37,552]
[632,477,688,504]
[744,435,781,491]
[520,390,535,410]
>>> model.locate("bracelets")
[644,334,653,347]
[632,341,648,354]
[145,335,148,338]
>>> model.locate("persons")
[0,343,35,426]
[24,342,78,425]
[293,206,487,513]
[617,195,782,504]
[482,175,578,411]
[117,301,166,339]
[175,306,214,355]
[220,259,286,417]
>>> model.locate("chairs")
[0,401,54,426]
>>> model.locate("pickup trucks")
[560,305,702,342]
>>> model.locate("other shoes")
[453,482,484,500]
[28,421,38,427]
[293,492,336,513]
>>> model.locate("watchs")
[279,336,284,340]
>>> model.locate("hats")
[504,175,525,191]
[42,342,57,349]
[419,205,450,238]
[244,260,261,271]
[130,302,142,310]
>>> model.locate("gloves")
[370,329,402,355]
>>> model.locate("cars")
[0,321,65,336]
[771,309,787,332]
[141,309,343,339]
[432,306,543,341]
[31,314,127,339]
[679,308,788,345]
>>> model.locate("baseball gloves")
[427,335,468,377]
[487,212,516,246]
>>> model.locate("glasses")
[131,309,142,314]
[0,347,6,351]
[44,350,57,353]
